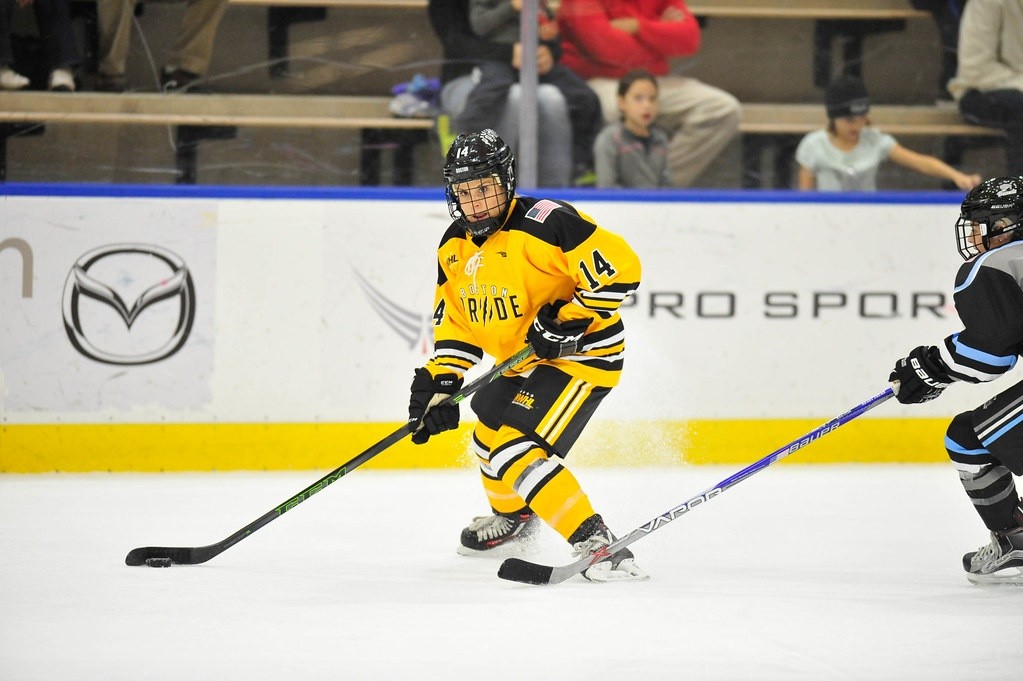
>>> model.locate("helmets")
[444,128,516,238]
[955,176,1023,261]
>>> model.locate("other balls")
[145,557,172,568]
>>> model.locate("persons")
[0,0,225,93]
[592,69,672,190]
[946,0,1023,177]
[428,0,602,188]
[408,128,651,584]
[794,74,980,191]
[888,174,1023,586]
[554,0,745,189]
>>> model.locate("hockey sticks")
[125,341,537,566]
[496,381,902,585]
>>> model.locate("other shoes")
[97,74,127,90]
[161,66,225,95]
[0,70,28,91]
[48,70,74,92]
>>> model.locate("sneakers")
[458,507,541,559]
[961,527,1023,586]
[566,515,650,583]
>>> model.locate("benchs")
[0,1,1016,189]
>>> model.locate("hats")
[825,75,869,117]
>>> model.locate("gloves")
[889,343,956,404]
[522,300,593,360]
[409,366,464,444]
